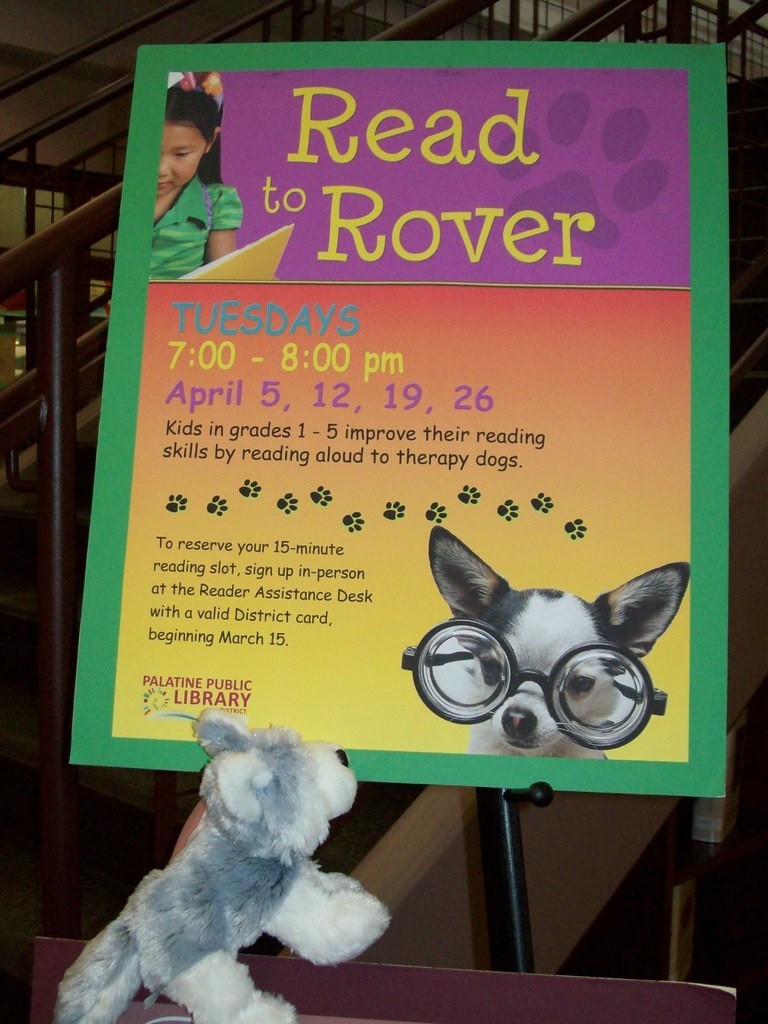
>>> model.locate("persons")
[146,80,244,286]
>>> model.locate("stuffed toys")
[56,708,393,1024]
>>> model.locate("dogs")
[427,525,690,760]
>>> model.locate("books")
[175,221,295,282]
